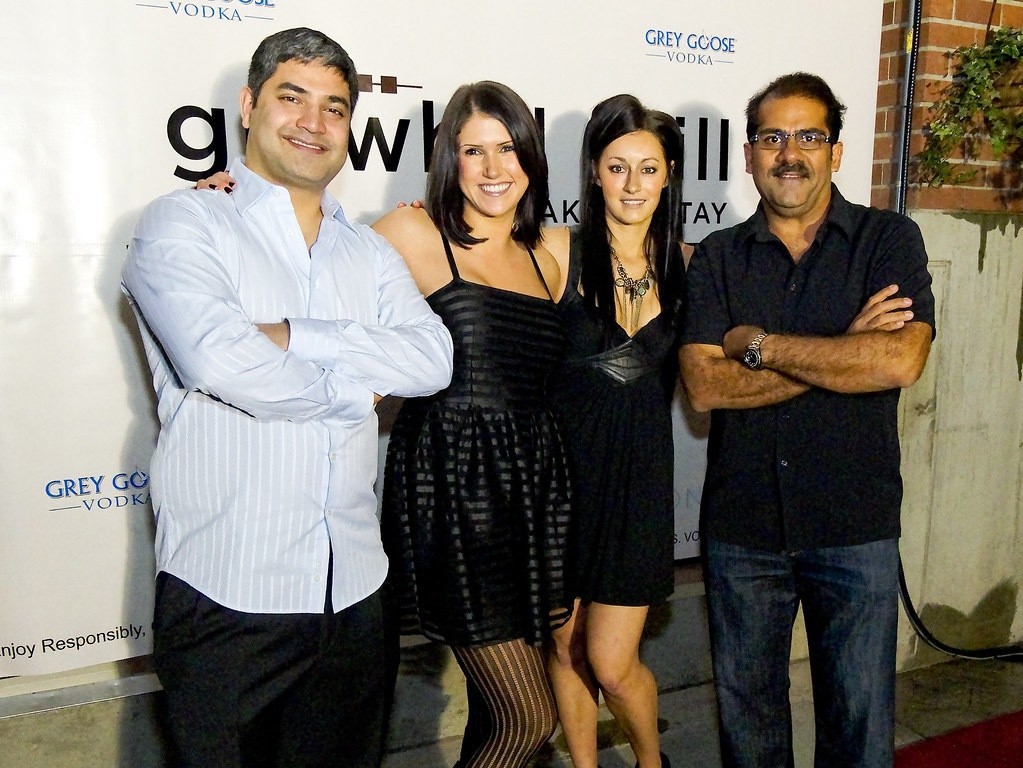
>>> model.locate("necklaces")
[611,246,652,300]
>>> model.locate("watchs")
[744,333,768,371]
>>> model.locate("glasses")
[749,131,835,149]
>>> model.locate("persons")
[674,71,935,768]
[395,94,695,768]
[193,81,581,768]
[120,26,455,768]
